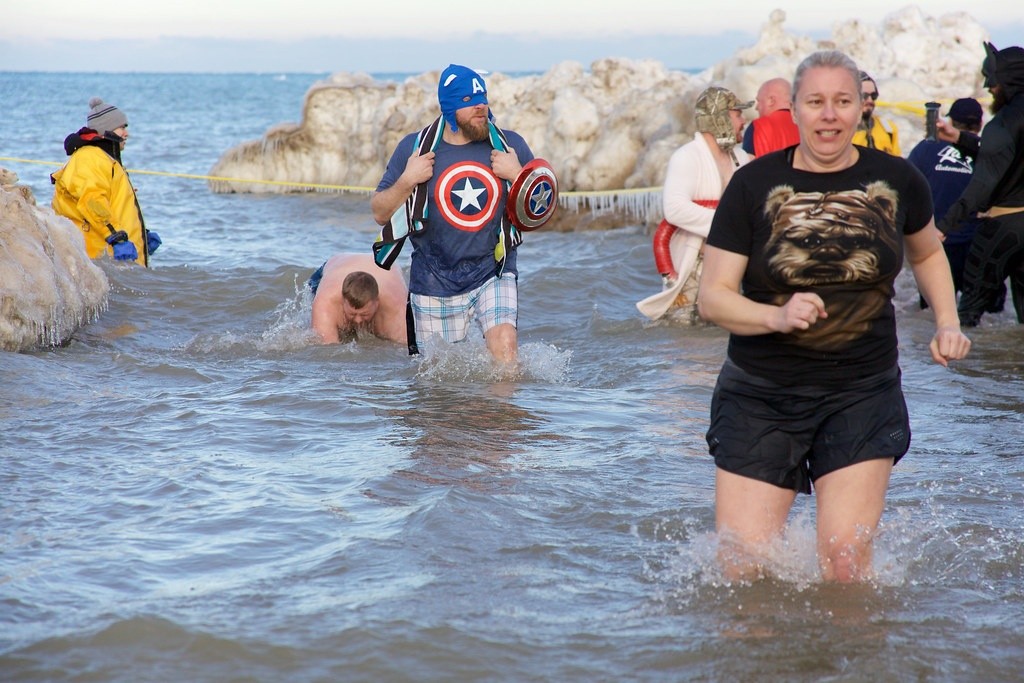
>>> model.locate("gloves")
[146,230,162,255]
[107,232,138,261]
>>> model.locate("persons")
[694,51,972,587]
[905,99,1005,317]
[51,98,163,268]
[743,78,804,159]
[924,41,1024,327]
[635,86,757,327]
[848,67,897,157]
[308,252,414,346]
[371,64,544,363]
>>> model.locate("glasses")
[861,92,878,100]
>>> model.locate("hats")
[86,98,127,136]
[438,64,496,133]
[694,87,756,167]
[981,41,1023,85]
[945,98,982,124]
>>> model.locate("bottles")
[925,102,941,142]
